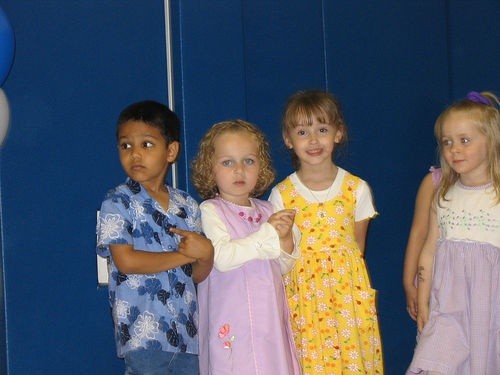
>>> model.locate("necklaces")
[305,178,332,207]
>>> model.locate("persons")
[191,119,304,375]
[96,100,215,375]
[268,88,385,375]
[402,91,500,375]
